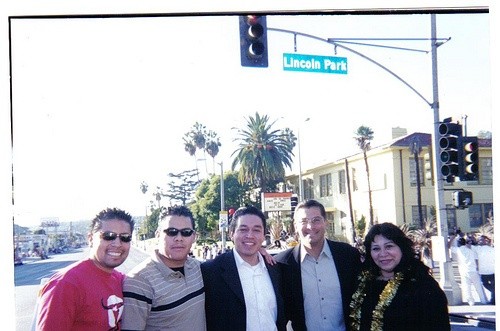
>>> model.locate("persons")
[272,200,365,331]
[33,207,135,331]
[420,227,495,306]
[120,204,207,331]
[200,204,289,331]
[347,222,451,331]
[274,230,298,252]
[200,243,230,260]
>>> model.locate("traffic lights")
[239,14,269,69]
[436,116,463,183]
[452,191,473,208]
[457,135,480,182]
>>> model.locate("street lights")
[297,117,311,201]
[196,157,226,253]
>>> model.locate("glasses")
[100,231,132,242]
[296,216,324,225]
[163,227,194,237]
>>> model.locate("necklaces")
[349,270,405,331]
